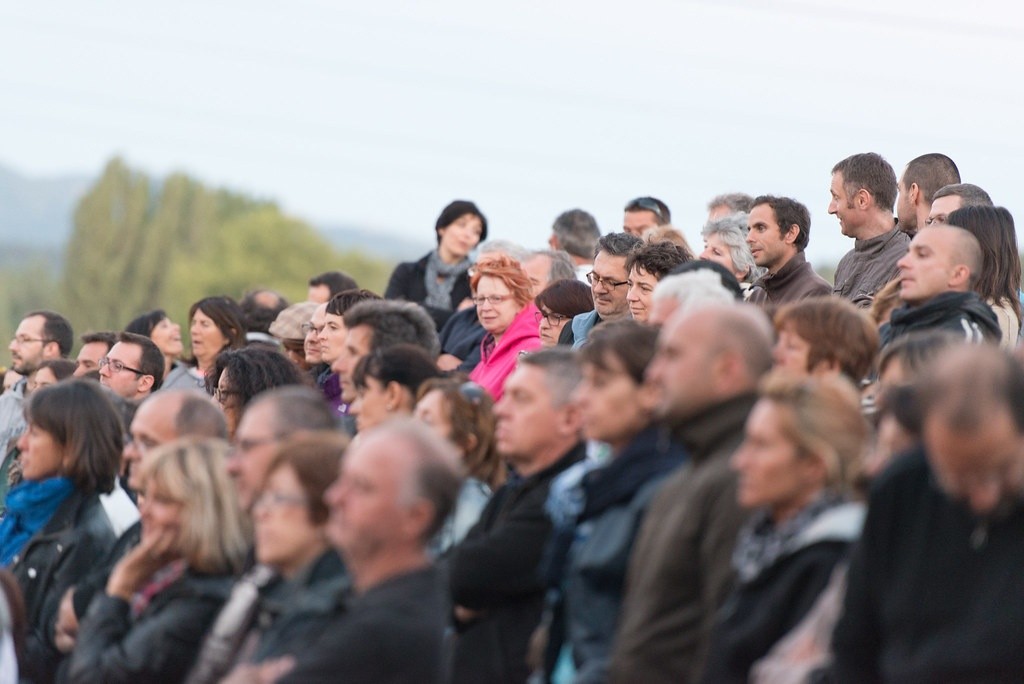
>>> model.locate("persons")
[855,224,1002,423]
[897,153,1024,351]
[701,298,880,684]
[748,332,1024,684]
[549,208,599,265]
[623,196,669,239]
[829,152,912,309]
[699,193,768,303]
[385,199,490,320]
[740,195,832,300]
[0,238,773,684]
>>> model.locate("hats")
[269,300,321,344]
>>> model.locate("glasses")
[624,199,665,224]
[925,213,949,224]
[99,360,148,376]
[473,294,515,306]
[9,335,63,349]
[460,380,486,432]
[587,271,627,292]
[284,345,304,357]
[868,385,924,433]
[301,324,324,335]
[213,388,239,403]
[535,311,574,326]
